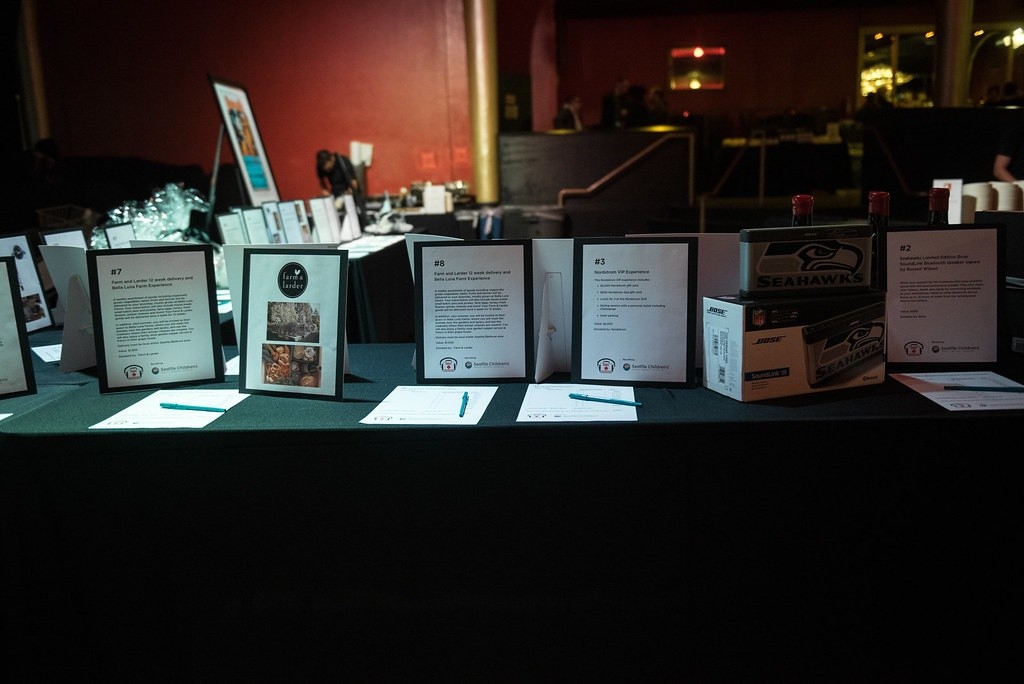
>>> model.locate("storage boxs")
[740,226,873,296]
[702,292,887,403]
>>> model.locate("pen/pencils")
[943,384,1024,393]
[159,402,226,412]
[568,393,643,407]
[458,391,470,417]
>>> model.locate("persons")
[550,74,671,133]
[14,136,71,229]
[857,77,1023,111]
[315,149,365,211]
[992,120,1023,183]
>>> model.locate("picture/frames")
[104,221,134,250]
[0,256,38,401]
[413,239,535,384]
[878,226,1005,372]
[214,190,362,252]
[0,231,56,333]
[39,223,93,260]
[84,242,225,396]
[238,244,351,402]
[209,77,281,209]
[570,235,690,384]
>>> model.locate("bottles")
[790,194,814,227]
[868,192,889,227]
[929,188,950,226]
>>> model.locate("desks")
[0,344,1024,683]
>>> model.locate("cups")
[962,181,1024,224]
